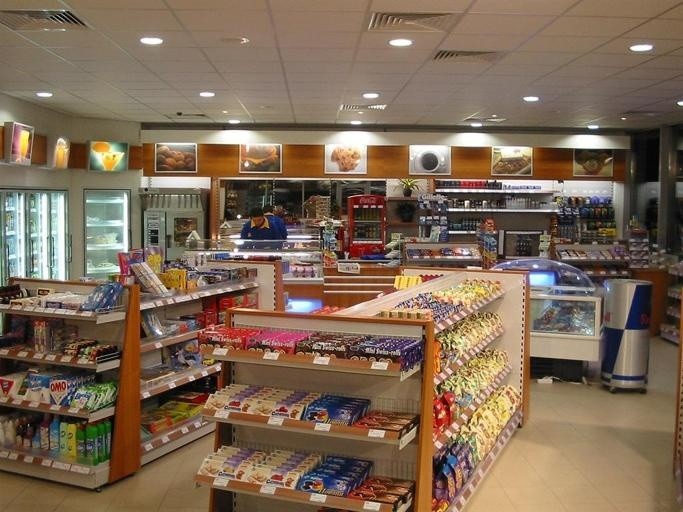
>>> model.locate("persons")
[240,206,288,250]
[262,204,287,248]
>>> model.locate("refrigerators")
[0,167,144,285]
[348,193,387,256]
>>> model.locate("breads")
[331,145,361,170]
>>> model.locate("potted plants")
[400,178,420,197]
[397,203,416,223]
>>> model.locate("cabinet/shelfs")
[427,178,561,260]
[1,259,282,493]
[185,238,324,304]
[530,285,605,380]
[387,196,419,227]
[660,259,683,346]
[195,265,530,511]
[549,243,632,284]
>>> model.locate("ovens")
[142,193,206,262]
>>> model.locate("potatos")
[156,147,195,170]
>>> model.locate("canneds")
[568,197,584,208]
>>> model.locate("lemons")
[93,142,110,151]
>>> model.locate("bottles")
[353,225,380,239]
[516,240,531,256]
[39,412,113,467]
